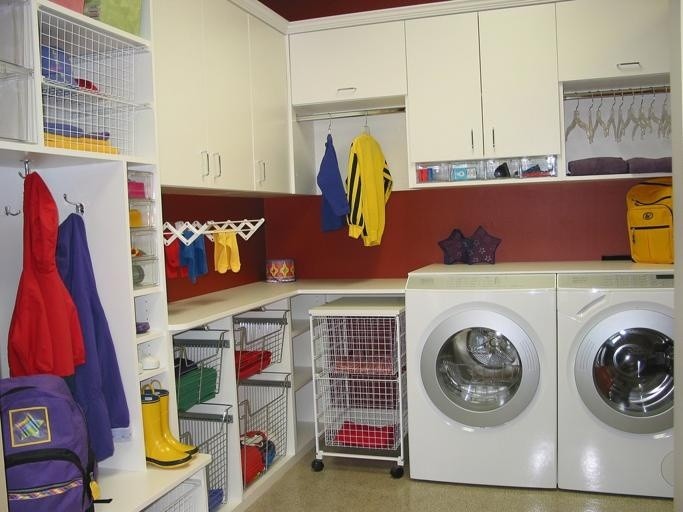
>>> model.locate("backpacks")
[625,177,675,264]
[1,376,113,508]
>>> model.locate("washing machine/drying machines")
[556,273,675,500]
[405,275,558,489]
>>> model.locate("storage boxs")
[317,373,407,449]
[39,8,135,100]
[138,339,162,363]
[135,292,163,331]
[170,324,230,414]
[315,314,395,376]
[180,402,233,512]
[132,260,158,287]
[238,371,291,490]
[130,201,154,228]
[128,170,154,199]
[42,79,135,153]
[232,307,291,376]
[453,165,477,181]
[130,231,156,257]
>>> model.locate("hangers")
[626,89,645,130]
[617,89,625,136]
[646,88,663,130]
[325,112,333,140]
[607,89,618,141]
[586,91,595,141]
[632,88,654,139]
[565,93,589,143]
[363,109,370,137]
[594,90,607,136]
[657,88,671,137]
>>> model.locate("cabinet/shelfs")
[407,0,562,188]
[554,0,683,180]
[151,0,251,190]
[286,7,408,190]
[0,0,212,512]
[246,0,292,193]
[168,280,407,512]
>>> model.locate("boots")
[140,385,191,468]
[142,380,199,458]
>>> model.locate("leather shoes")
[137,323,149,333]
